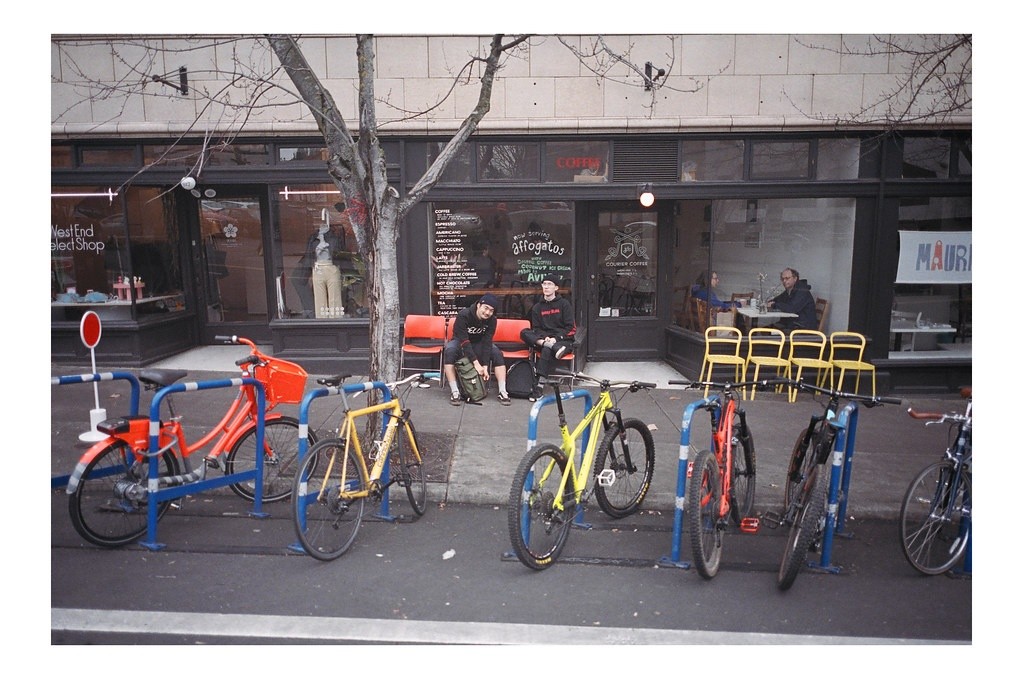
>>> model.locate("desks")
[433,286,572,315]
[735,305,799,335]
[889,320,957,352]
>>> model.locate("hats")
[542,274,560,286]
[480,293,499,308]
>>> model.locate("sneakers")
[450,391,462,406]
[496,391,511,406]
[528,385,544,402]
[551,343,566,359]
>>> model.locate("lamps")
[181,167,200,190]
[636,183,655,207]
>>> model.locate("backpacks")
[506,358,537,399]
[455,356,488,406]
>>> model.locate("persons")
[298,224,345,319]
[455,242,496,308]
[479,203,512,287]
[444,294,511,406]
[520,275,574,401]
[761,268,818,335]
[691,270,746,328]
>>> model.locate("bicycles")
[506,368,660,572]
[770,377,906,590]
[899,385,972,576]
[499,272,654,319]
[66,334,320,547]
[667,379,795,581]
[290,370,444,563]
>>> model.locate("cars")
[73,194,350,245]
[623,220,657,254]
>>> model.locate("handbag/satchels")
[710,302,736,338]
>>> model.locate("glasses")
[541,284,556,287]
[780,275,794,281]
[712,277,718,281]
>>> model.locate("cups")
[740,299,747,307]
[750,298,759,309]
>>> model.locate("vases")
[759,298,768,314]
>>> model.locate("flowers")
[755,272,778,305]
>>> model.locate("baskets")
[255,356,308,405]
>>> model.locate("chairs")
[672,285,875,402]
[400,313,575,393]
[937,342,972,351]
